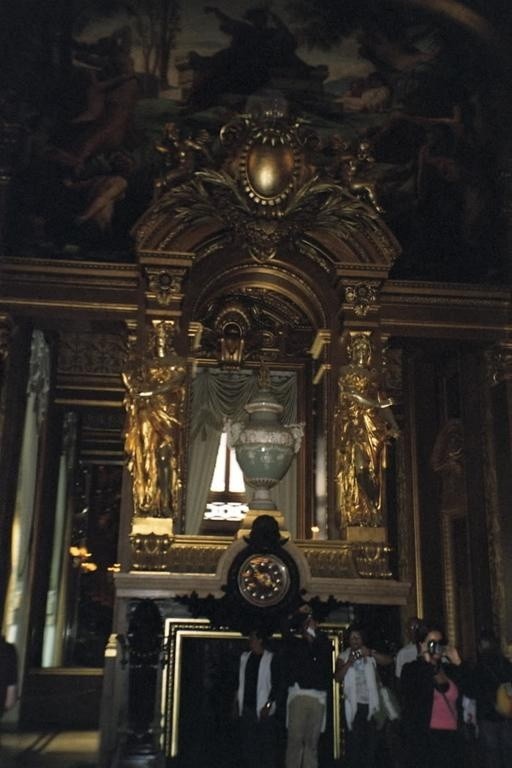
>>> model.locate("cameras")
[428,638,449,655]
[351,647,362,664]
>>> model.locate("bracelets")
[429,655,440,663]
[367,647,372,657]
[265,702,271,706]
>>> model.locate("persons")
[277,602,334,768]
[334,333,402,528]
[120,321,185,520]
[471,627,511,768]
[0,632,24,717]
[229,625,281,768]
[392,615,451,681]
[497,630,511,698]
[333,622,402,768]
[397,622,482,768]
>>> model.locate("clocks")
[236,552,292,607]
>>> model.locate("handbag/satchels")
[377,685,402,721]
